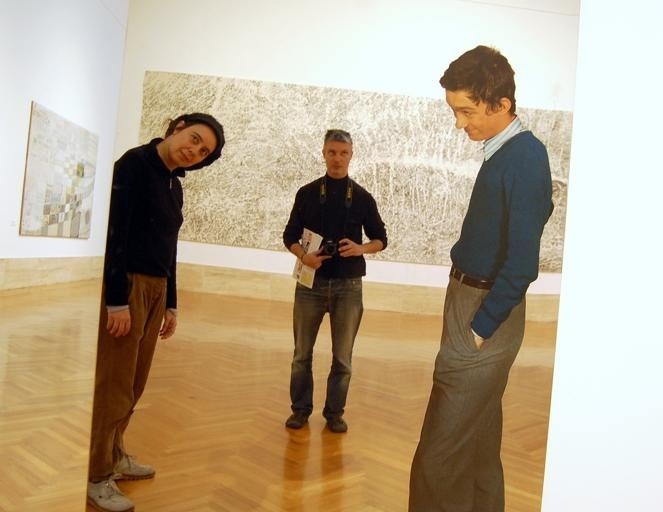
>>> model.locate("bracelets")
[299,253,305,264]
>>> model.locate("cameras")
[319,241,342,257]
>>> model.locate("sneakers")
[88,480,134,511]
[112,456,155,479]
[286,412,309,428]
[327,416,347,432]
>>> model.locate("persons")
[283,130,387,432]
[82,113,224,512]
[409,45,554,512]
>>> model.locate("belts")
[450,265,495,289]
